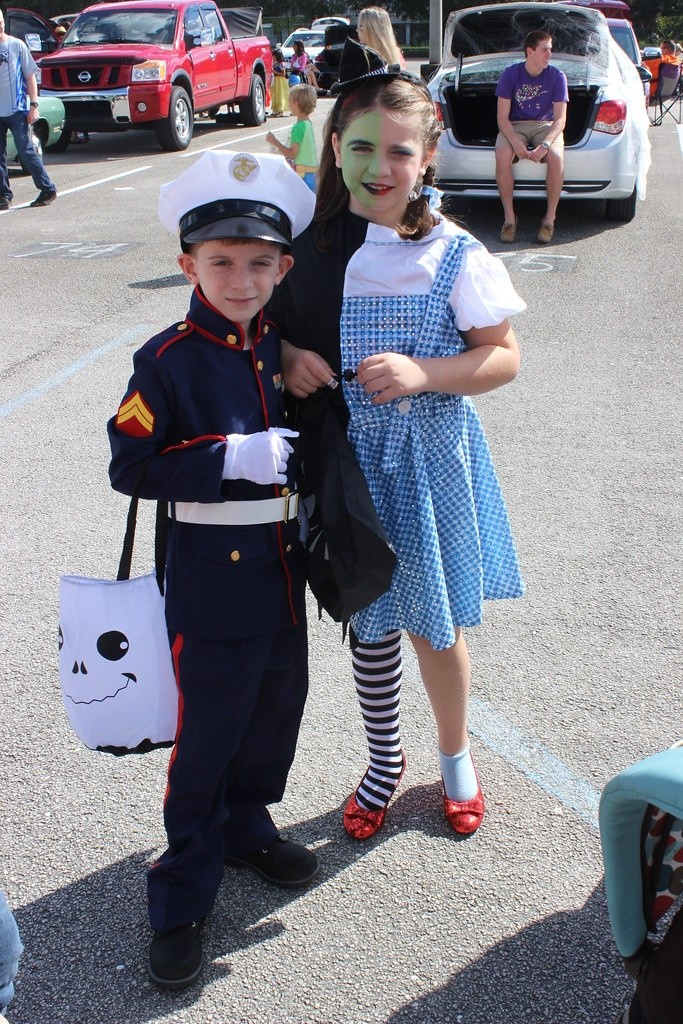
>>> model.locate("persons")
[109,151,322,990]
[199,39,325,119]
[46,19,90,144]
[642,40,683,108]
[260,65,527,844]
[0,9,57,211]
[265,84,320,190]
[495,30,571,244]
[357,7,407,69]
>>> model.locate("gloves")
[222,427,299,485]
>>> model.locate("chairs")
[646,62,683,126]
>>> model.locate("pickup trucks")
[5,0,273,152]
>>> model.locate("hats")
[156,150,317,254]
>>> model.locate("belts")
[167,488,302,525]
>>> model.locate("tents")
[1,6,56,49]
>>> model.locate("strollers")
[598,740,682,1024]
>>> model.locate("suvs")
[275,17,351,77]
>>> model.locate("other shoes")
[442,750,485,834]
[148,917,206,989]
[274,111,283,117]
[220,834,320,887]
[344,750,406,838]
[537,216,554,243]
[30,190,57,207]
[501,215,517,243]
[0,198,9,210]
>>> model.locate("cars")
[426,2,652,223]
[314,25,358,90]
[49,14,98,43]
[643,47,661,57]
[6,95,65,175]
[560,0,650,110]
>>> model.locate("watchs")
[30,102,39,107]
[541,141,550,151]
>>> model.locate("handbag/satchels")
[58,452,180,757]
